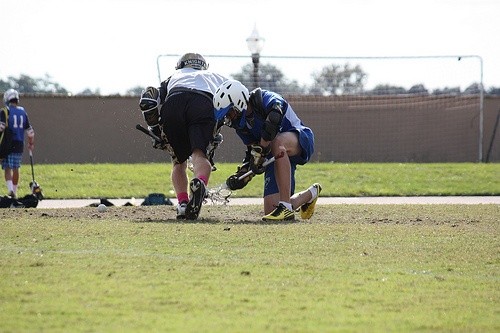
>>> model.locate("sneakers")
[300,182,322,220]
[176,200,188,219]
[185,177,205,220]
[262,203,294,221]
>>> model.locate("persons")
[0,89,34,199]
[138,52,224,219]
[213,80,322,221]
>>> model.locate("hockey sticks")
[209,151,285,204]
[135,122,230,205]
[29,148,43,200]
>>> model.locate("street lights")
[246,27,265,89]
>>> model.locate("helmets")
[212,79,249,130]
[174,52,207,70]
[3,89,19,105]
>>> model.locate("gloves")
[248,144,270,175]
[210,134,223,147]
[148,124,168,150]
[28,144,33,150]
[226,166,251,190]
